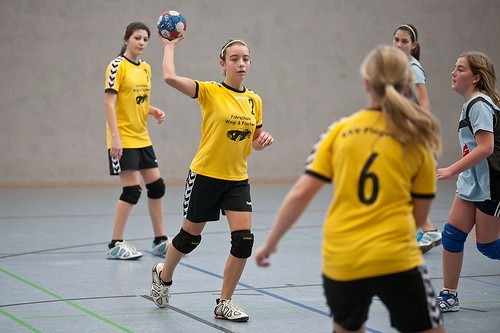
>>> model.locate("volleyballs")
[156,10,187,40]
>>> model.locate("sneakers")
[104,240,143,260]
[436,290,461,312]
[151,262,173,308]
[213,297,250,321]
[151,240,172,259]
[417,227,444,254]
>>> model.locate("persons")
[393,24,441,254]
[436,51,500,312]
[150,29,273,321]
[104,22,171,260]
[255,45,444,333]
